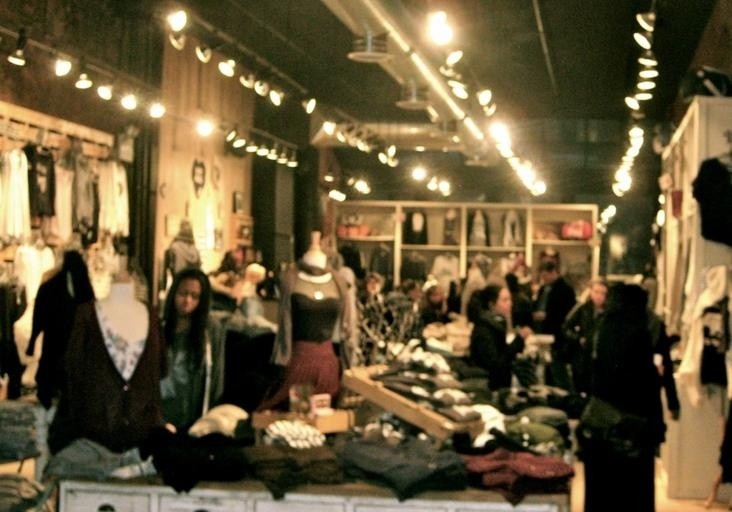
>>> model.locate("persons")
[582,283,681,512]
[209,251,274,315]
[158,266,225,426]
[0,281,26,399]
[272,233,351,399]
[64,272,167,447]
[357,262,608,393]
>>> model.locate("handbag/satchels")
[576,396,655,462]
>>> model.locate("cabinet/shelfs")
[331,201,599,287]
